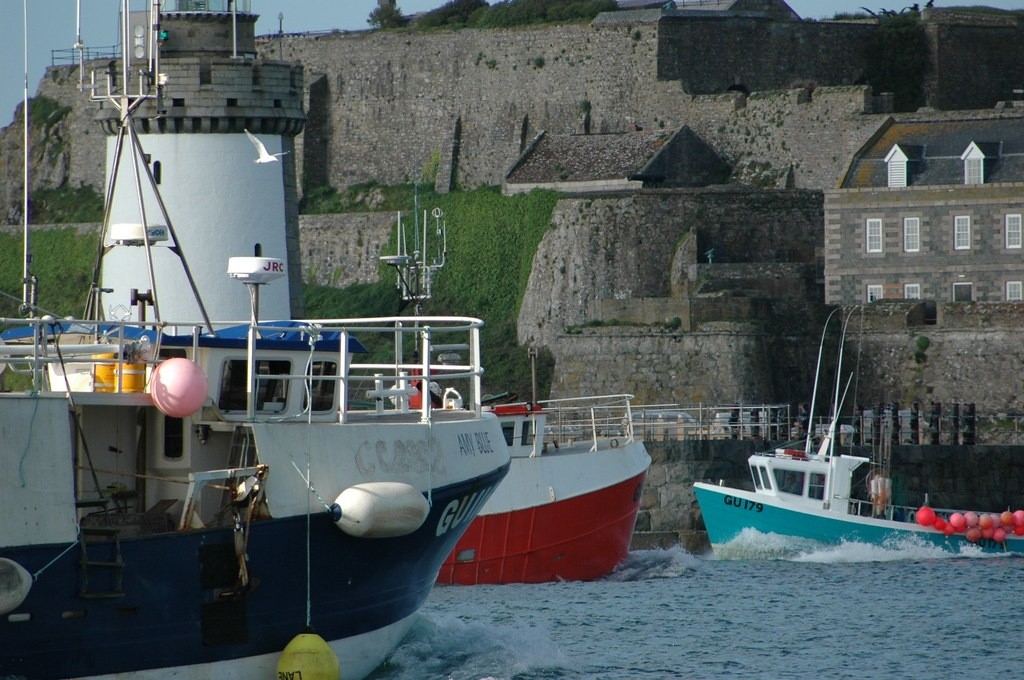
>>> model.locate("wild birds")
[244,128,290,164]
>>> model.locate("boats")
[0,0,514,680]
[690,303,1024,566]
[342,184,651,589]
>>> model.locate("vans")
[622,411,699,436]
